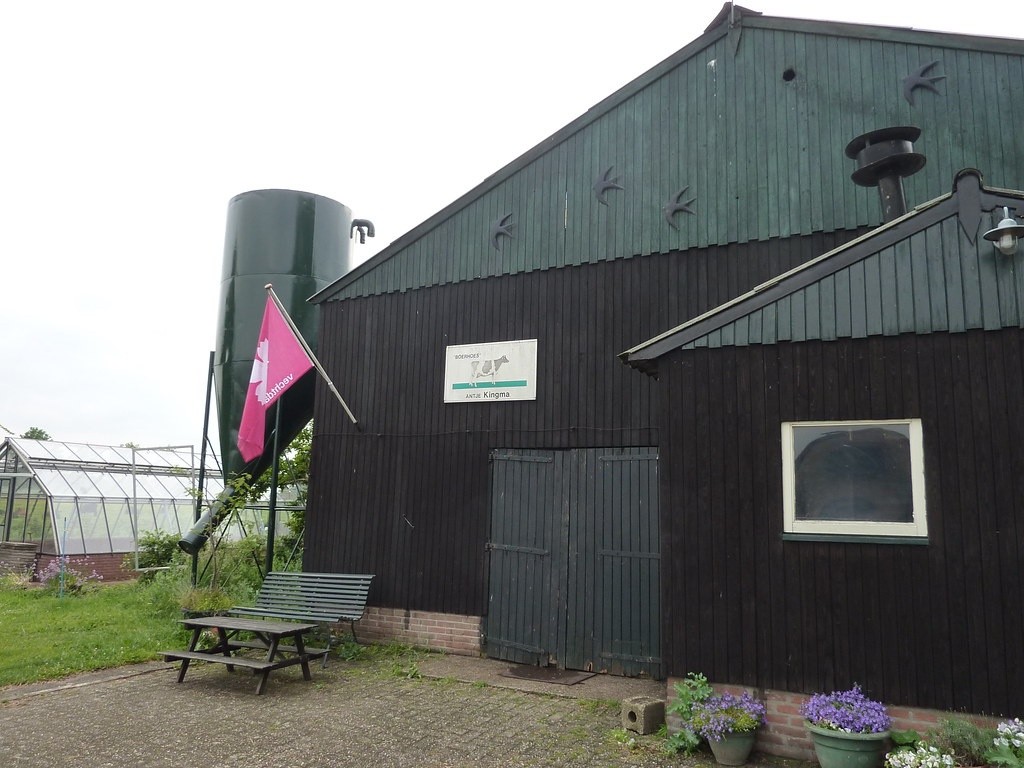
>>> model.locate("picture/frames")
[680,691,769,741]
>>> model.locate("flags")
[236,294,314,463]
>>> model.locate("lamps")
[982,206,1024,254]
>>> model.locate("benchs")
[227,639,331,681]
[157,650,279,695]
[228,570,376,669]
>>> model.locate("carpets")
[497,667,597,686]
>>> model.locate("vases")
[803,720,892,768]
[707,729,756,766]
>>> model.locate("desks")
[175,616,318,695]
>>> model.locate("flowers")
[885,717,1024,768]
[799,682,895,733]
